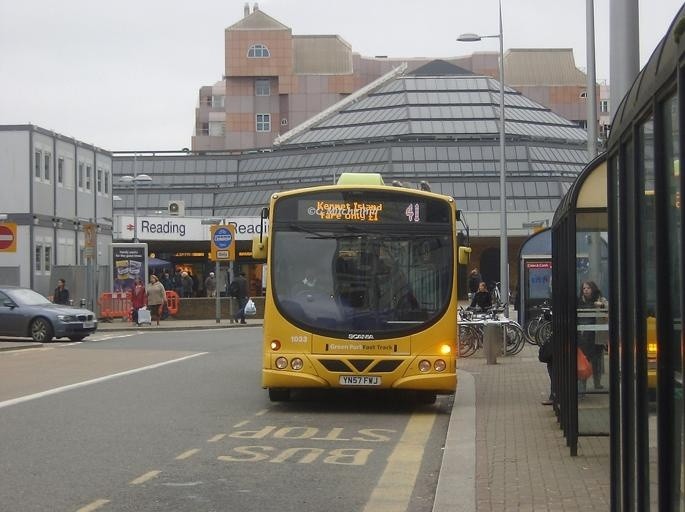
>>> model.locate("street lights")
[455,31,512,318]
[119,172,152,243]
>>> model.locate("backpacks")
[229,280,240,297]
[538,338,552,363]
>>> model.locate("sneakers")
[594,383,604,389]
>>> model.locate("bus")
[641,188,685,398]
[257,171,473,404]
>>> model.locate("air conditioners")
[168,199,185,216]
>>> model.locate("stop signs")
[0,223,16,254]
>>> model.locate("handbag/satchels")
[577,346,593,383]
[137,309,152,325]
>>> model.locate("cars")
[0,284,98,344]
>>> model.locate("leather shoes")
[235,318,248,324]
[541,400,556,405]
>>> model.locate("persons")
[466,281,493,315]
[574,282,608,396]
[467,266,482,305]
[130,267,250,326]
[539,309,554,405]
[52,279,69,304]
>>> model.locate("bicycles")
[486,278,512,302]
[527,301,554,349]
[453,304,525,357]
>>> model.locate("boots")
[157,316,161,326]
